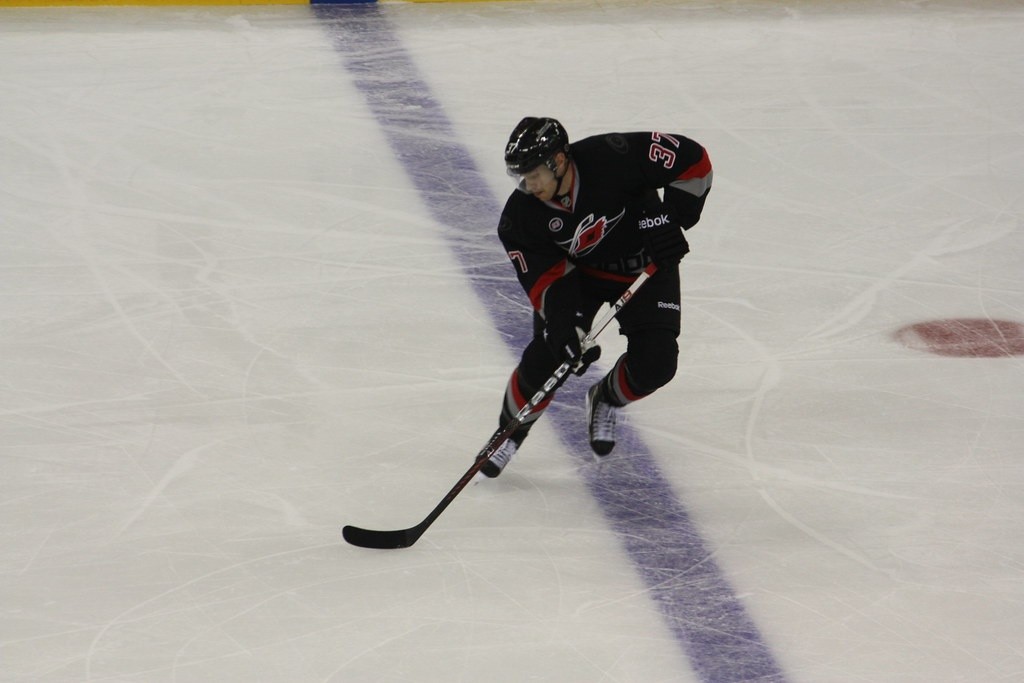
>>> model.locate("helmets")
[505,117,570,175]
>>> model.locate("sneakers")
[476,428,528,478]
[586,378,617,458]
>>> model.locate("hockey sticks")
[340,260,657,551]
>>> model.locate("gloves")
[640,213,688,270]
[544,308,601,377]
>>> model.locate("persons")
[475,117,714,485]
